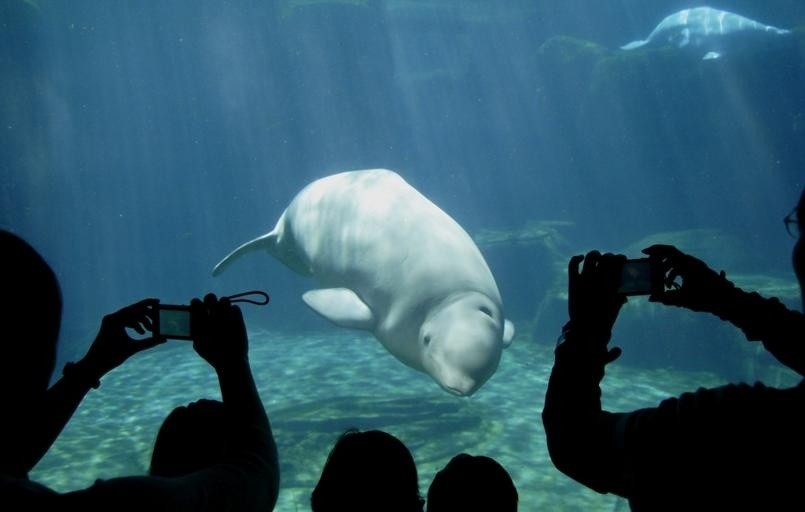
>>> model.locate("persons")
[427,449,523,510]
[143,394,225,476]
[309,428,426,511]
[0,227,282,509]
[540,181,803,510]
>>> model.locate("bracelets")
[55,355,104,395]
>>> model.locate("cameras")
[623,257,665,297]
[153,303,193,340]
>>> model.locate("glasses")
[784,209,802,239]
